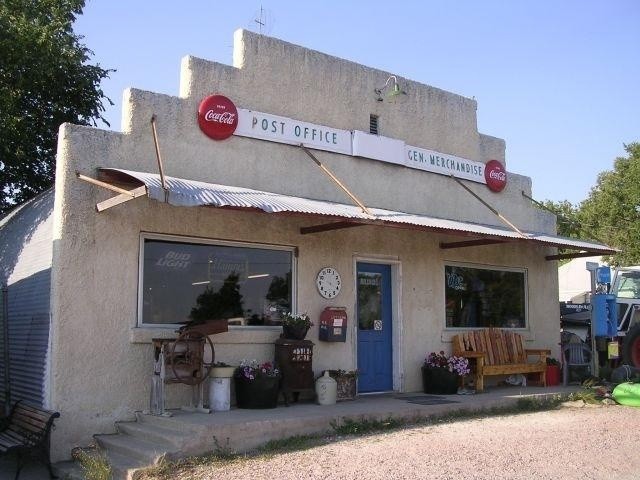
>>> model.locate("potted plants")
[546,357,562,386]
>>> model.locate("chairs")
[560,342,593,387]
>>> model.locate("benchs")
[451,327,552,392]
[0,398,61,479]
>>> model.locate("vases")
[421,367,460,395]
[235,375,280,410]
[283,324,308,340]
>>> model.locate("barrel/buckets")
[589,293,619,337]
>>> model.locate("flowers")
[279,311,314,330]
[421,350,471,377]
[235,357,281,384]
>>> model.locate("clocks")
[314,266,342,300]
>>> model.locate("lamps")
[373,74,409,106]
